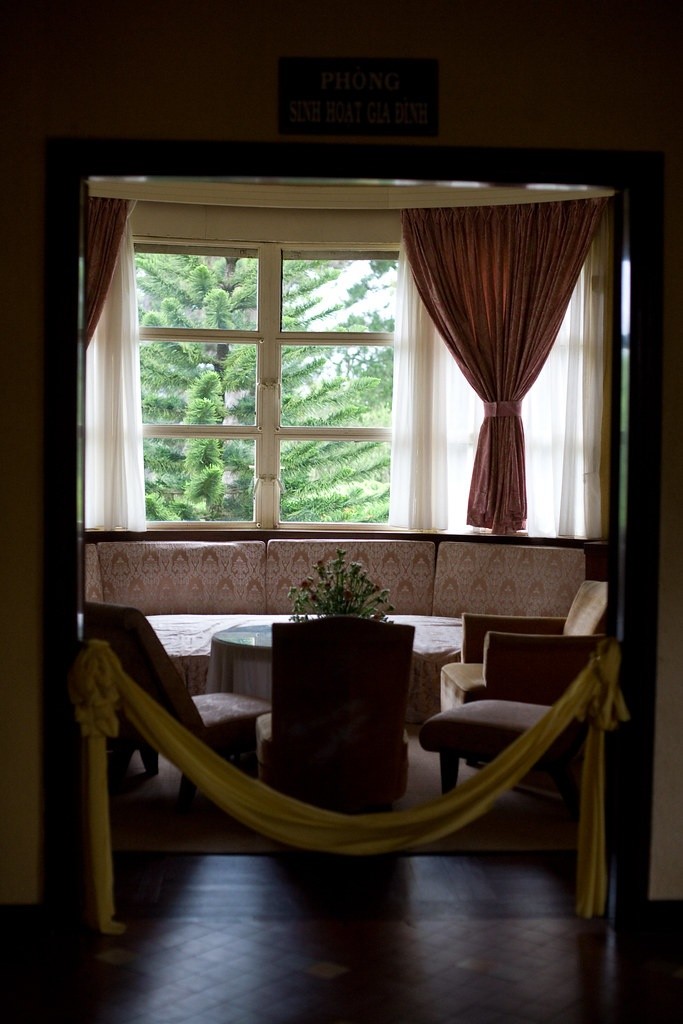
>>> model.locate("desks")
[207,624,276,703]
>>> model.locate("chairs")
[419,641,627,832]
[440,581,619,767]
[83,600,272,811]
[255,615,416,813]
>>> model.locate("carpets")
[107,723,577,853]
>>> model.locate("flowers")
[286,551,399,624]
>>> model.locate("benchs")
[81,530,609,729]
